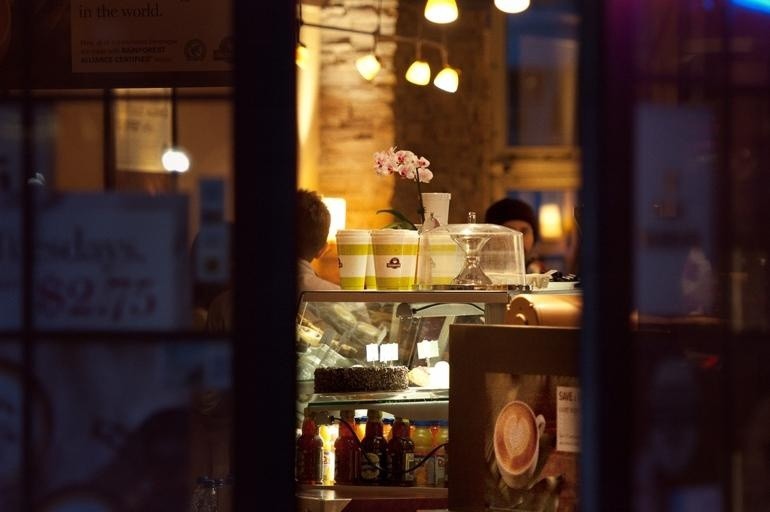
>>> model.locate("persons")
[484,199,538,274]
[206,188,373,344]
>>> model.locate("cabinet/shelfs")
[299,288,581,512]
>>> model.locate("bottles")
[296,405,448,488]
[187,178,236,334]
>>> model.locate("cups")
[337,229,377,291]
[371,229,419,292]
[422,191,452,227]
[492,400,547,491]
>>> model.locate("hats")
[484,198,540,246]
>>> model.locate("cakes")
[315,365,410,396]
